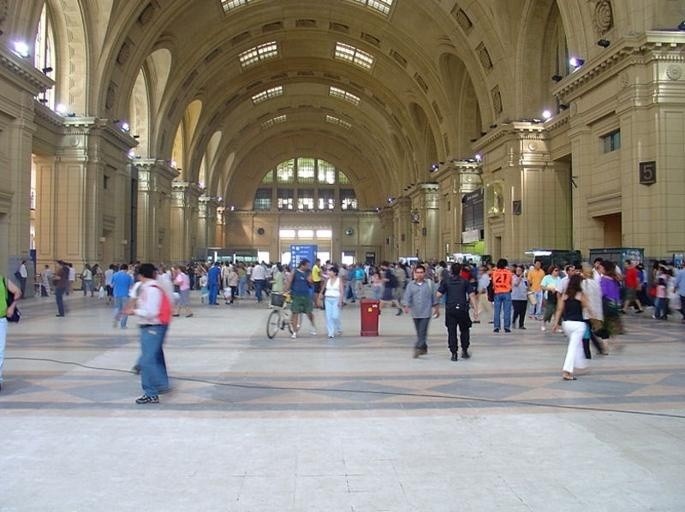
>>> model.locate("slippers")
[563,374,579,381]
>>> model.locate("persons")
[0,275,23,389]
[312,259,490,361]
[157,260,317,338]
[125,263,171,404]
[552,275,595,380]
[486,259,685,358]
[43,260,142,328]
[19,260,28,297]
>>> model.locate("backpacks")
[441,269,449,279]
[390,273,402,288]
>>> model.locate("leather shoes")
[171,313,194,318]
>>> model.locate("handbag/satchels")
[667,291,682,310]
[582,323,592,340]
[486,280,494,302]
[159,293,173,325]
[602,295,617,317]
[2,276,22,323]
[543,288,559,305]
[320,287,327,310]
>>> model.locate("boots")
[460,350,471,358]
[451,353,457,361]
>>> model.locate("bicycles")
[264,290,304,341]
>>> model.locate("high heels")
[342,297,404,316]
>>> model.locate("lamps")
[570,58,584,68]
[552,75,562,82]
[43,67,52,76]
[597,39,610,48]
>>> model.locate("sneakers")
[414,344,428,358]
[209,299,265,306]
[136,386,171,404]
[290,329,343,338]
[617,309,685,323]
[473,315,564,333]
[56,295,128,330]
